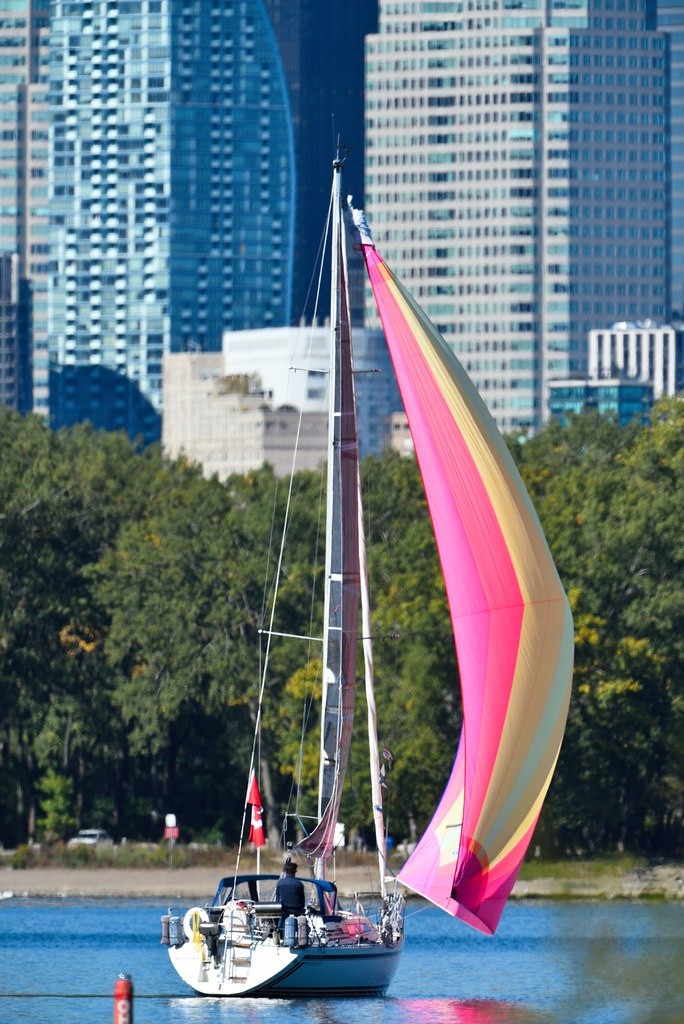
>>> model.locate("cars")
[66,829,111,847]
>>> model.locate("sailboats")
[166,110,577,1001]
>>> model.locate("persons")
[277,863,306,940]
